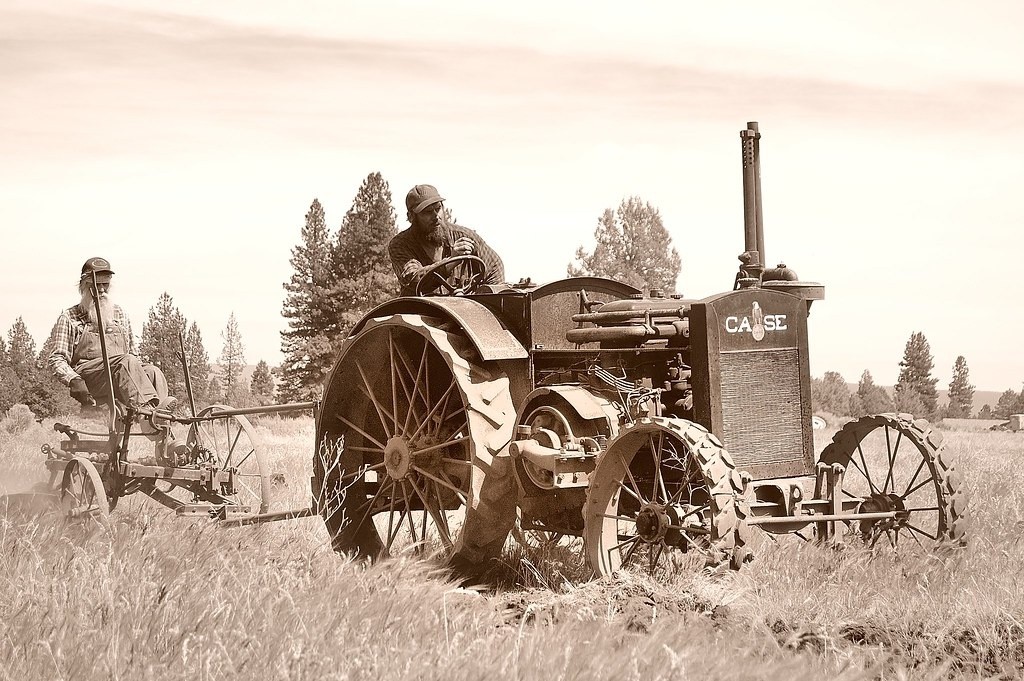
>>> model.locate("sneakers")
[155,436,186,467]
[138,396,178,440]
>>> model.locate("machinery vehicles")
[308,121,970,585]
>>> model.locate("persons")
[386,183,506,300]
[48,256,188,467]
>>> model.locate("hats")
[81,257,115,278]
[406,184,446,213]
